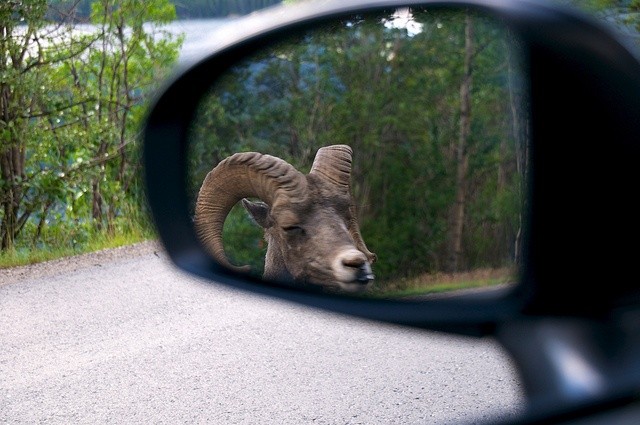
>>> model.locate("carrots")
[194,144,378,301]
